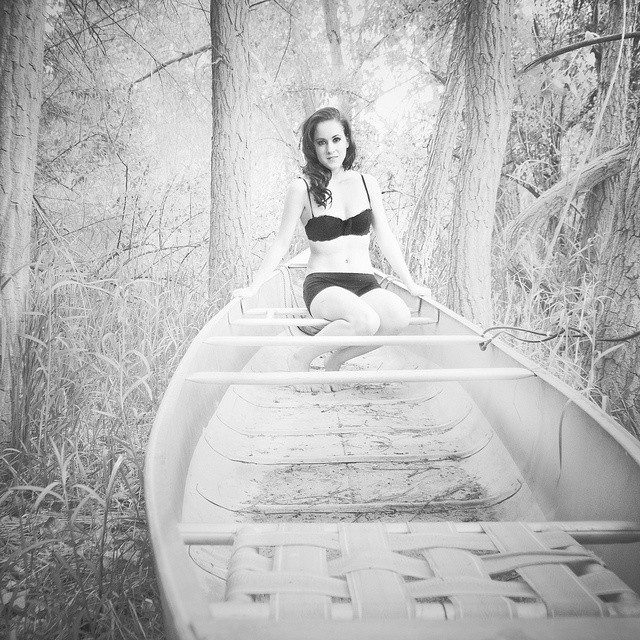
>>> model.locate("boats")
[145,252,640,636]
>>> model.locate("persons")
[232,108,431,393]
